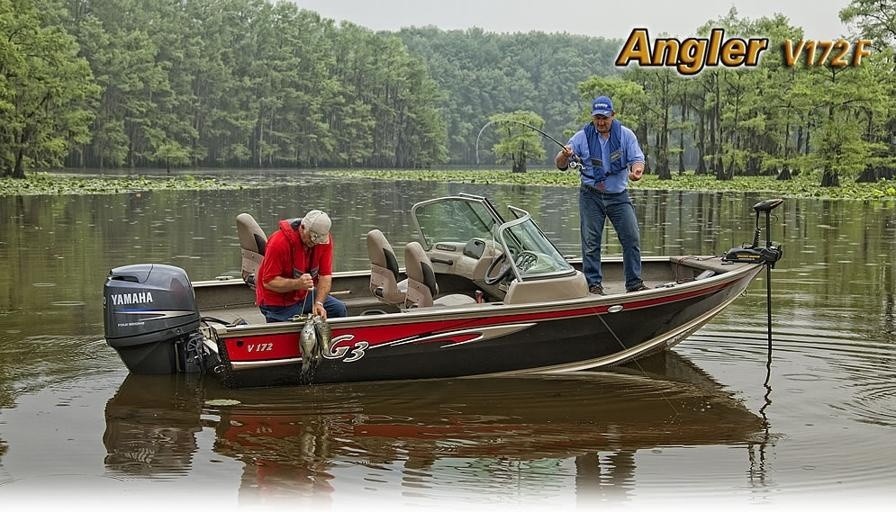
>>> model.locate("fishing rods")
[199,290,351,310]
[476,119,583,165]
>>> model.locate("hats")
[590,95,615,119]
[300,208,332,246]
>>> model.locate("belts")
[583,183,627,197]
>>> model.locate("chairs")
[366,229,440,305]
[404,242,477,309]
[235,213,268,291]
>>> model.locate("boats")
[101,192,785,390]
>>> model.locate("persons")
[254,209,348,322]
[555,96,651,294]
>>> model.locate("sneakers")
[589,284,608,297]
[626,280,653,292]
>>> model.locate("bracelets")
[315,300,323,307]
